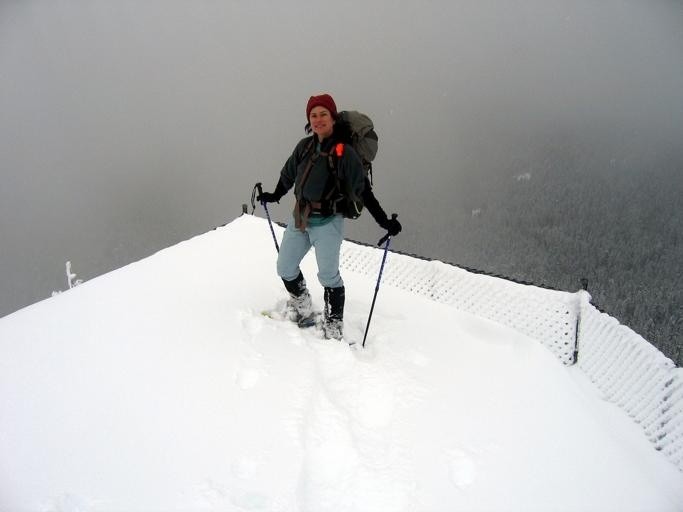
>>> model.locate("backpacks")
[297,110,378,219]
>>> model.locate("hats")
[306,94,336,121]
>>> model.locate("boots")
[323,287,345,339]
[282,272,315,327]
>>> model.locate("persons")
[256,93,403,343]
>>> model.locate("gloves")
[257,192,277,205]
[382,216,401,237]
